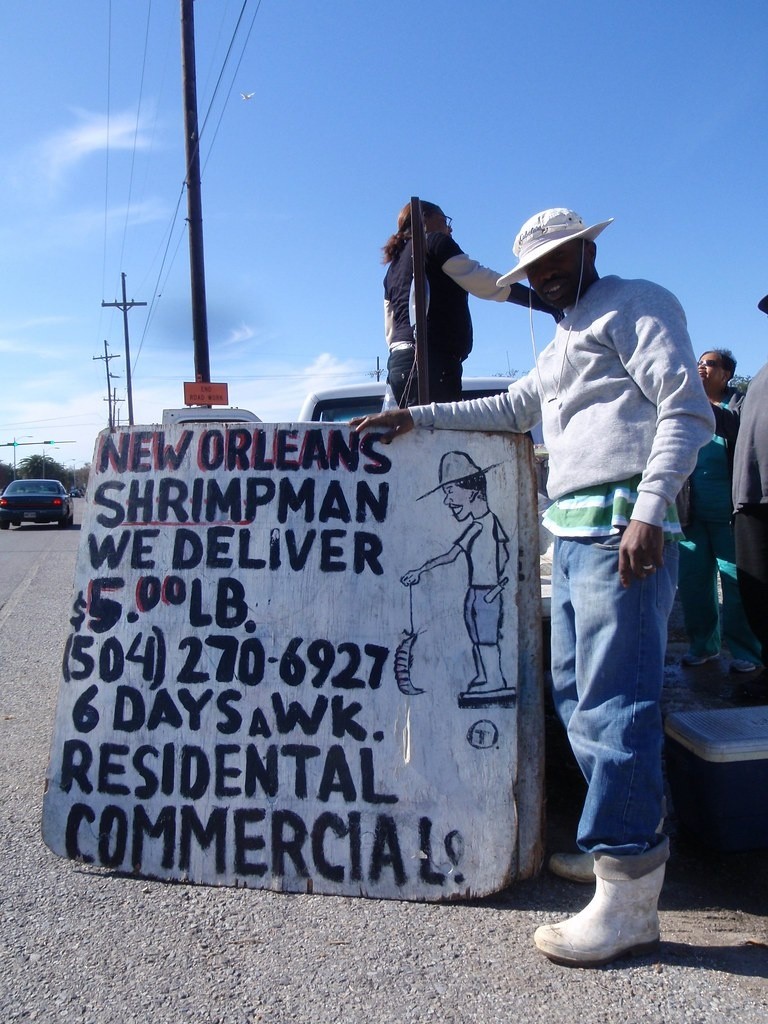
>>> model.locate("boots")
[531,832,670,966]
[547,796,669,883]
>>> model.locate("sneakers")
[681,651,720,666]
[728,658,758,672]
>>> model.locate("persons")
[349,207,714,965]
[732,296,768,659]
[383,201,564,410]
[678,349,757,671]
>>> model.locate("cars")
[0,479,74,530]
[68,489,84,498]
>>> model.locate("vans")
[163,377,551,621]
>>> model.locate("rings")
[642,564,653,570]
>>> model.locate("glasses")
[697,360,723,367]
[434,209,454,229]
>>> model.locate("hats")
[757,294,768,315]
[496,207,615,288]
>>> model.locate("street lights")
[63,459,76,469]
[43,447,60,479]
[74,461,86,487]
[14,435,33,481]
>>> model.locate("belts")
[390,343,414,353]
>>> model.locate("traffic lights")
[7,442,17,446]
[43,441,53,444]
